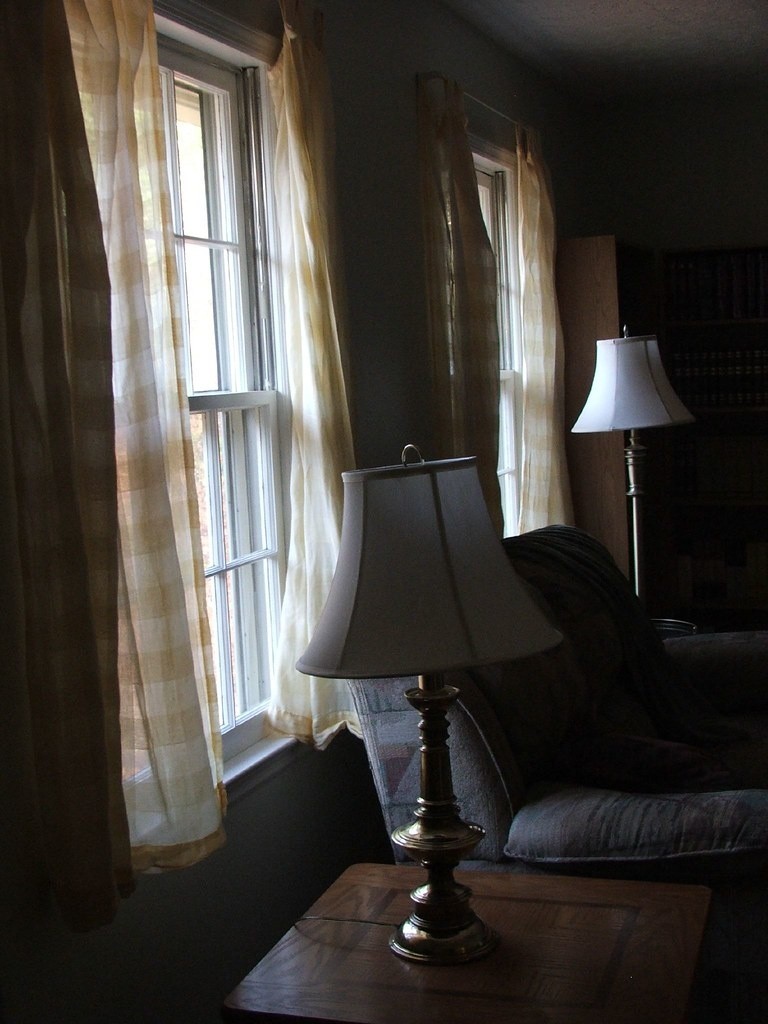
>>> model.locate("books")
[664,251,768,610]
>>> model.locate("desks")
[223,863,715,1024]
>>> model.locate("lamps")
[302,443,565,969]
[570,323,694,597]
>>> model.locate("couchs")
[353,525,768,890]
